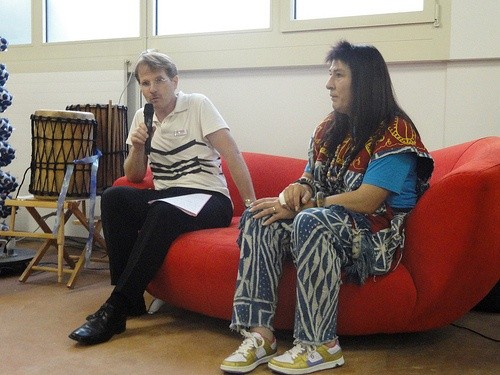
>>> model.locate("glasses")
[140,76,169,90]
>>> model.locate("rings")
[273,207,276,214]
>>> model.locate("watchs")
[243,199,253,207]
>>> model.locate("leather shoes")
[68,302,126,344]
[86,295,147,321]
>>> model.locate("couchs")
[111,137,500,333]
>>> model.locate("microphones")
[143,104,154,155]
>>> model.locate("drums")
[65,103,129,196]
[27,109,99,200]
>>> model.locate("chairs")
[0,197,107,289]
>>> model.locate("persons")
[219,40,434,375]
[68,50,257,345]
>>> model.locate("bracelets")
[314,192,327,208]
[294,177,316,197]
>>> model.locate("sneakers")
[268,339,344,375]
[220,329,277,373]
